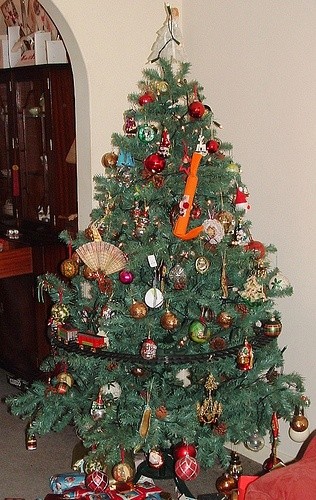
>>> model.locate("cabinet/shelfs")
[1,63,78,383]
